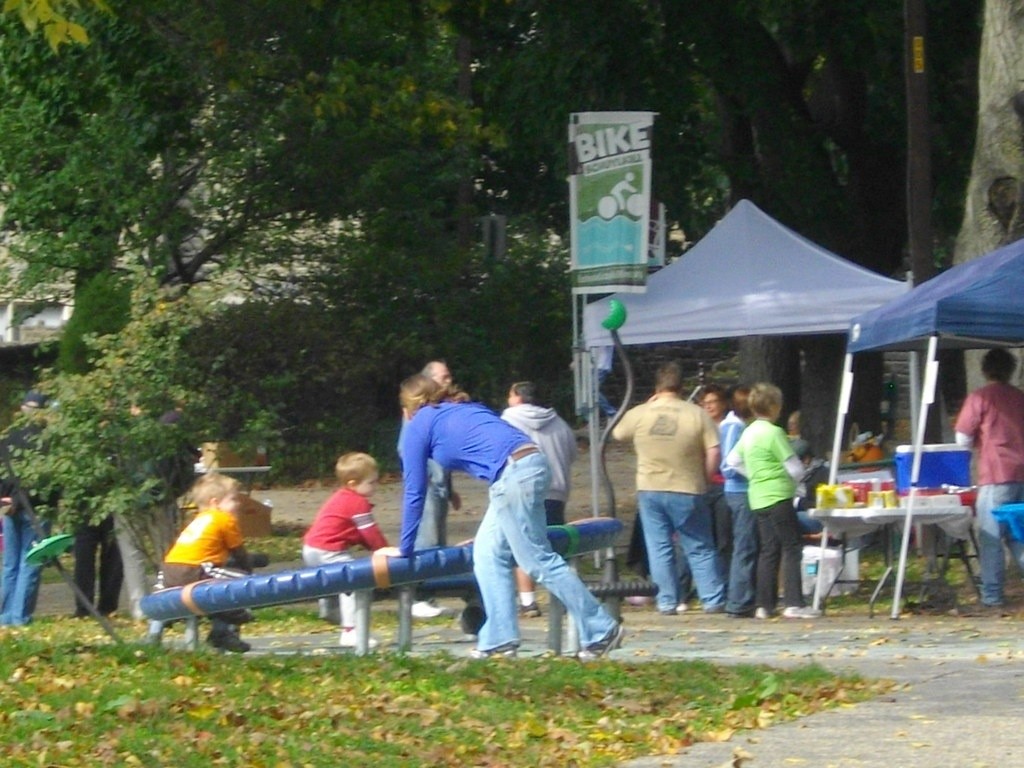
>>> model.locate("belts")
[504,448,540,464]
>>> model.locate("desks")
[839,459,894,470]
[805,504,991,617]
[196,464,273,496]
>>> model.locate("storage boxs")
[239,493,273,537]
[842,482,872,502]
[801,545,844,596]
[899,494,963,507]
[898,489,978,506]
[894,443,973,494]
[881,481,894,491]
[816,484,856,508]
[867,490,898,507]
[201,441,242,467]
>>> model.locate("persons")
[397,360,470,618]
[373,375,623,659]
[0,384,202,629]
[499,381,579,618]
[954,348,1024,611]
[629,378,832,618]
[303,452,390,647]
[609,361,726,613]
[162,474,254,652]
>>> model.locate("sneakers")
[470,643,518,658]
[205,628,250,653]
[208,608,255,623]
[578,625,623,661]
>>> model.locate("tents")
[810,237,1024,621]
[583,199,922,571]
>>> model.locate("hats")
[22,388,51,409]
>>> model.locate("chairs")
[802,531,832,542]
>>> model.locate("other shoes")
[412,601,442,618]
[625,596,650,606]
[726,610,756,618]
[957,601,1005,616]
[660,609,676,615]
[784,605,823,619]
[675,603,689,612]
[755,607,781,619]
[339,628,378,648]
[515,602,542,618]
[706,607,725,614]
[317,597,335,624]
